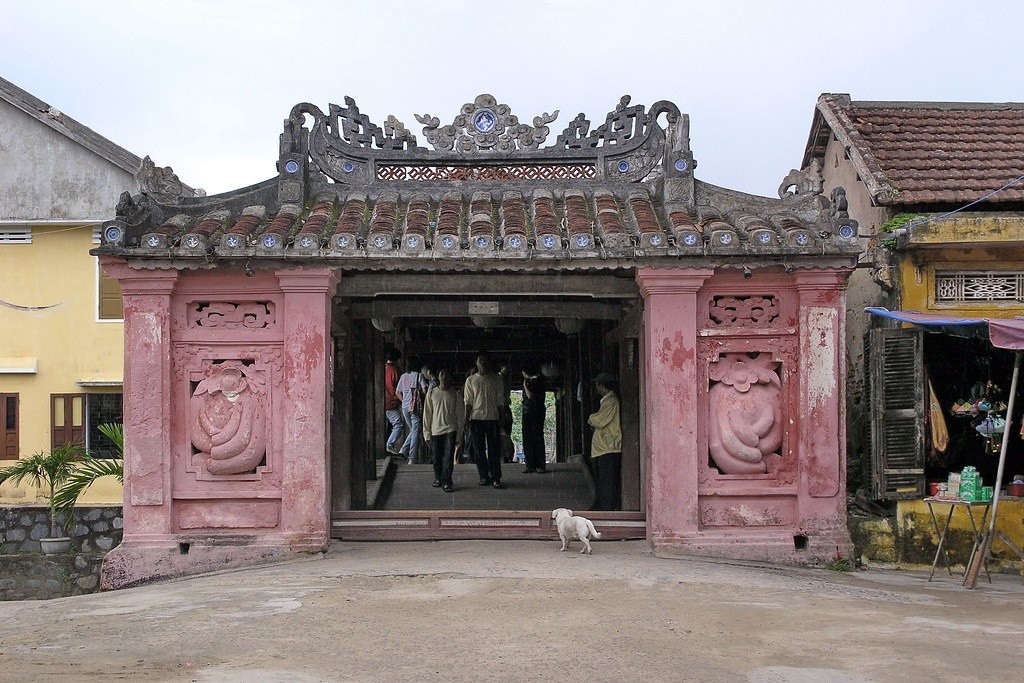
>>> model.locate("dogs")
[552,508,601,555]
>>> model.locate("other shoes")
[386,449,398,456]
[398,450,408,459]
[408,459,414,466]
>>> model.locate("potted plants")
[0,434,96,558]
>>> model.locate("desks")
[924,497,992,587]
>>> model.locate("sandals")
[522,466,534,473]
[478,479,491,485]
[432,479,442,487]
[536,467,545,473]
[493,481,501,488]
[442,484,453,492]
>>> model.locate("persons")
[423,365,464,492]
[462,352,502,488]
[522,366,546,473]
[385,352,518,465]
[587,373,622,511]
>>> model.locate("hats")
[592,373,615,388]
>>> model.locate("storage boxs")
[1007,484,1024,497]
[944,468,994,501]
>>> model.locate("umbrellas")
[863,306,1024,528]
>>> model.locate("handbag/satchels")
[407,372,425,417]
[927,377,950,457]
[499,428,514,458]
